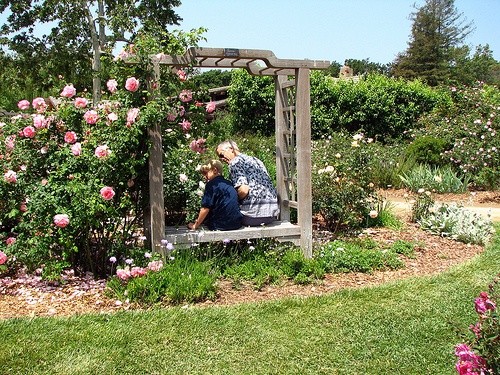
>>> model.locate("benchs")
[145,220,314,265]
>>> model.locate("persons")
[186,158,243,231]
[216,140,279,227]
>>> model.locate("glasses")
[220,153,224,158]
